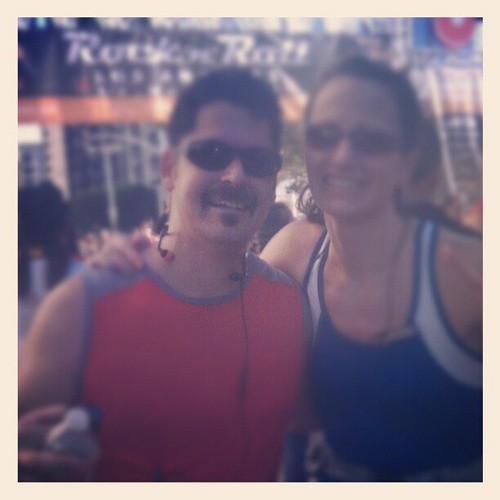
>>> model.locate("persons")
[19,169,486,334]
[19,62,315,478]
[84,52,482,481]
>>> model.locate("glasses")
[305,122,410,159]
[171,139,283,178]
